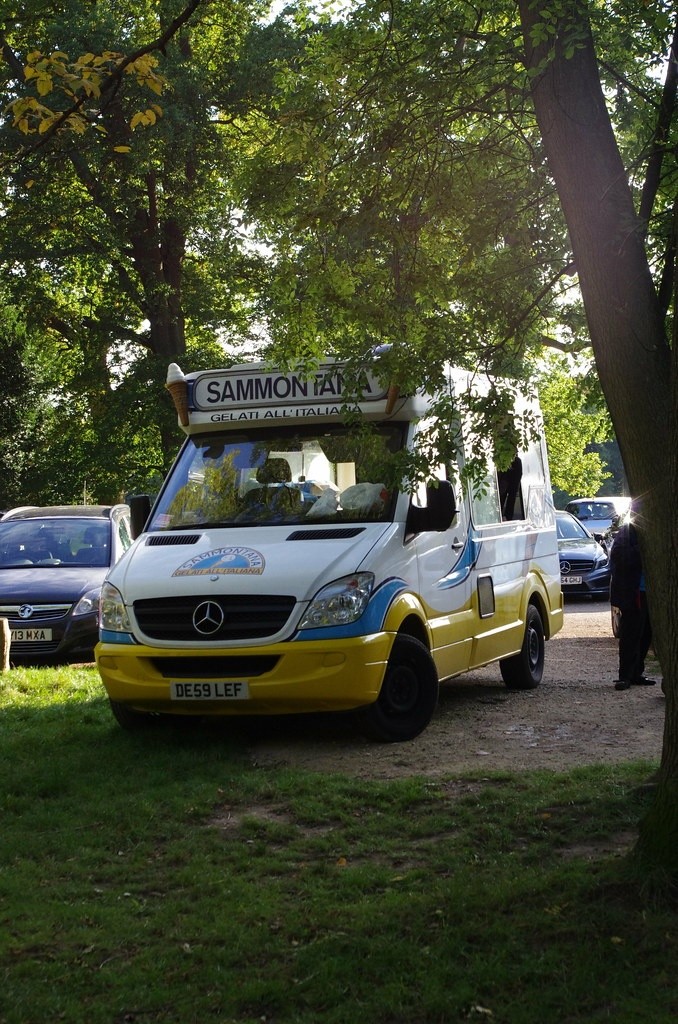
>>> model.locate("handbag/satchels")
[339,482,390,520]
[306,488,338,518]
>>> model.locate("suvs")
[1,502,130,664]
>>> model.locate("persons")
[33,527,72,562]
[609,505,657,691]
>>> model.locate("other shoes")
[630,674,656,685]
[613,677,630,690]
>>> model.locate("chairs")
[73,527,110,566]
[238,459,303,518]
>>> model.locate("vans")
[93,343,564,744]
[562,495,632,561]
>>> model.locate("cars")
[554,511,613,603]
